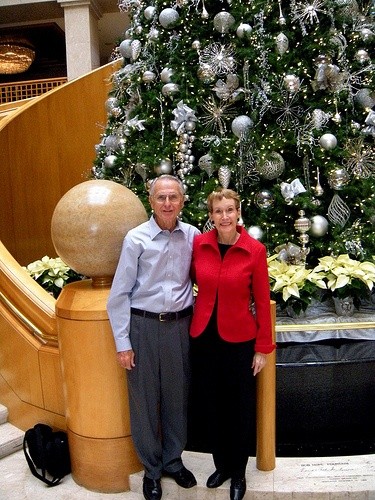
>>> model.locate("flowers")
[268,259,318,315]
[309,253,375,294]
[23,256,76,299]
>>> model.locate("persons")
[188,188,275,500]
[107,175,257,500]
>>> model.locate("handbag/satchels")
[22,424,72,487]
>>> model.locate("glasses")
[155,193,179,202]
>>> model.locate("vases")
[332,296,355,316]
[286,298,304,319]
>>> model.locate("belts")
[131,305,193,321]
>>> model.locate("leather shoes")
[230,478,246,500]
[162,466,197,488]
[142,474,162,500]
[207,469,231,488]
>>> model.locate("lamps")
[0,44,36,75]
